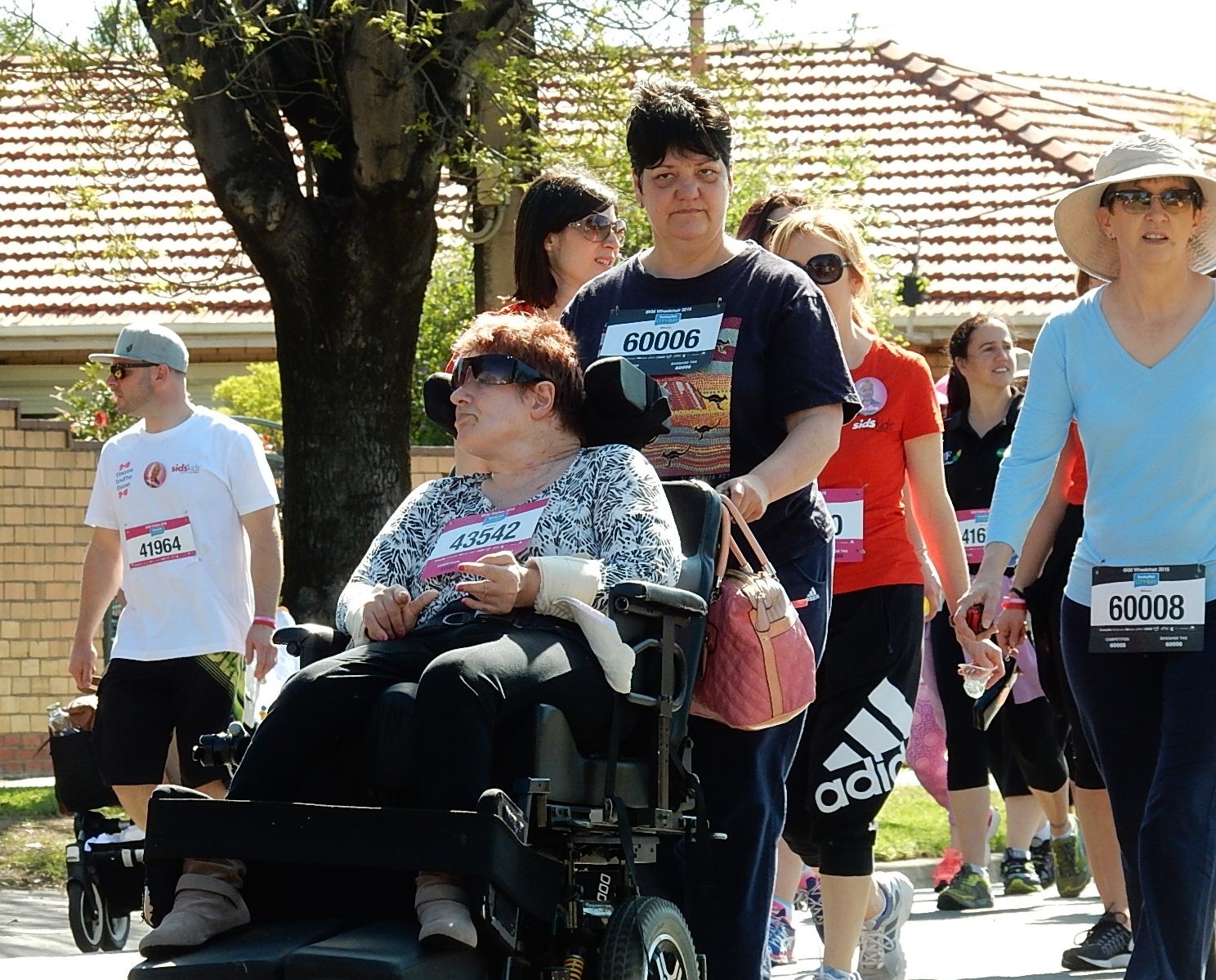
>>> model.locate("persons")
[954,134,1216,980]
[138,311,684,959]
[69,322,283,832]
[561,84,863,980]
[445,171,628,477]
[772,203,1005,980]
[734,191,825,968]
[899,315,1090,913]
[998,268,1135,970]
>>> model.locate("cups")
[958,662,998,699]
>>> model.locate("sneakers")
[1029,836,1055,890]
[931,849,963,893]
[810,957,862,980]
[797,870,825,944]
[999,847,1041,894]
[767,897,799,966]
[937,862,994,910]
[855,870,914,980]
[1061,902,1134,970]
[984,806,1001,870]
[1050,814,1091,898]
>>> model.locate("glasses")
[788,252,855,286]
[1105,188,1201,215]
[451,353,557,412]
[762,224,778,235]
[110,362,160,380]
[567,214,628,247]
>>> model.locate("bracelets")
[1000,602,1028,610]
[253,620,275,629]
[1010,588,1025,599]
[254,615,276,624]
[1002,595,1026,604]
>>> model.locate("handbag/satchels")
[689,493,818,731]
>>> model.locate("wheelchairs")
[127,357,720,980]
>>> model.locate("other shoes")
[411,876,479,951]
[139,858,250,957]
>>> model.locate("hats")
[1053,129,1216,283]
[88,323,189,373]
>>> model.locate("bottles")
[47,700,73,737]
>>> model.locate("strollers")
[49,732,147,952]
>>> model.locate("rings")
[484,581,488,595]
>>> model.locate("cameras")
[967,603,994,635]
